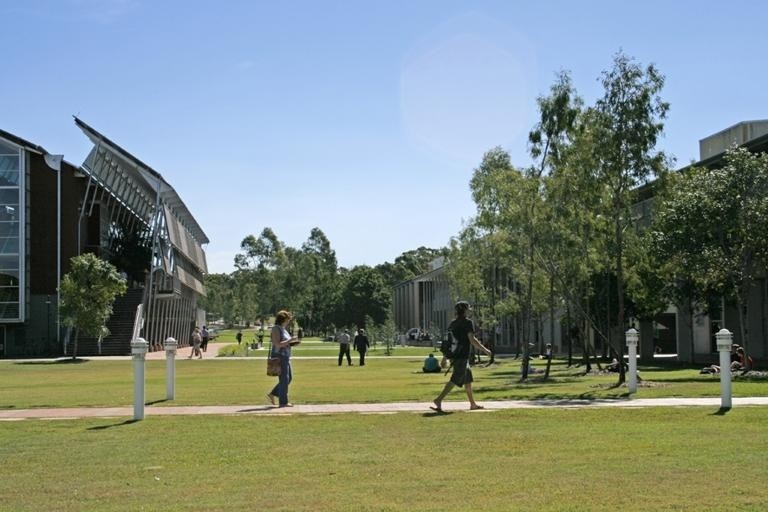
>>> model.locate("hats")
[289,337,300,346]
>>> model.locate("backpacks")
[440,319,458,358]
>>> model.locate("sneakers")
[266,393,294,407]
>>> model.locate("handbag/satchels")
[267,357,282,376]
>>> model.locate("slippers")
[430,406,444,412]
[469,405,484,409]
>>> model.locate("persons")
[237,331,243,345]
[265,309,295,407]
[429,301,492,414]
[543,344,552,359]
[606,358,619,372]
[257,328,264,343]
[187,324,210,360]
[731,346,753,369]
[709,343,739,372]
[416,327,431,341]
[423,353,441,372]
[354,328,369,366]
[337,329,354,366]
